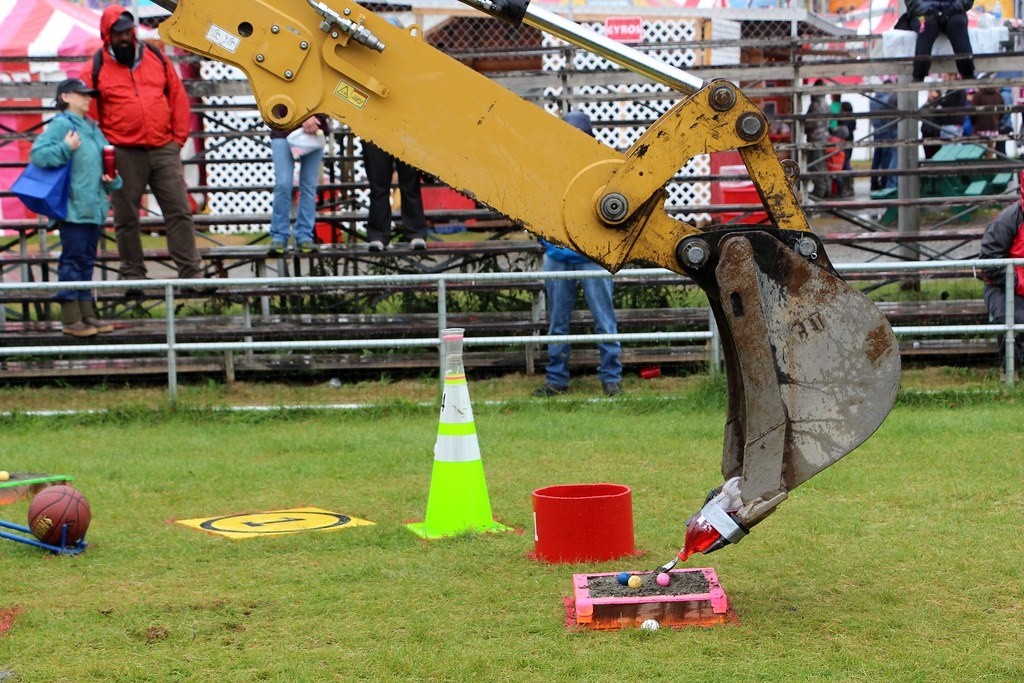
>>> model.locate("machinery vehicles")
[148,1,900,558]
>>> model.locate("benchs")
[0,24,1023,383]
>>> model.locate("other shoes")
[1000,370,1020,383]
[125,289,146,297]
[602,382,627,399]
[180,285,219,295]
[529,384,570,400]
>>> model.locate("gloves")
[932,6,956,31]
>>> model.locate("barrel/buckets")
[533,484,635,564]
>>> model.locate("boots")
[81,300,115,333]
[61,299,97,338]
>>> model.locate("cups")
[443,355,468,385]
[441,327,465,357]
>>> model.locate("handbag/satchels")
[7,158,71,222]
[286,125,327,160]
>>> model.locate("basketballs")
[28,486,91,546]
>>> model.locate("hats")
[109,12,139,32]
[55,78,101,100]
[563,109,596,137]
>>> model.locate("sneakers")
[267,241,290,256]
[369,240,397,253]
[296,241,319,253]
[408,239,431,251]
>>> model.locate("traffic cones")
[404,375,517,537]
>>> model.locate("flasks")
[103,145,117,179]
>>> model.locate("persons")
[805,78,854,199]
[30,77,123,338]
[358,141,426,251]
[83,5,220,296]
[532,112,624,397]
[979,170,1024,371]
[904,0,975,81]
[921,73,1024,158]
[870,78,898,190]
[267,114,330,257]
